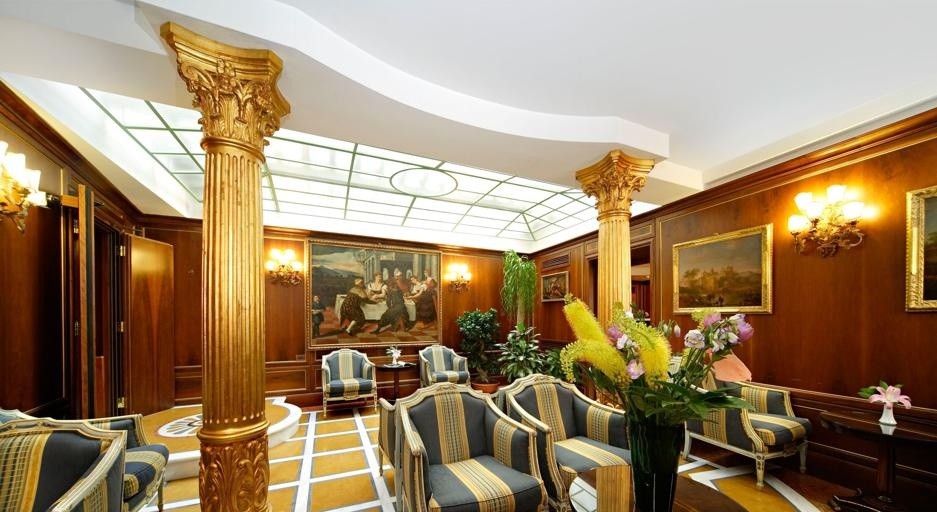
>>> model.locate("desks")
[374,362,418,405]
[817,406,937,512]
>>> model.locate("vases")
[630,423,681,511]
[878,407,897,436]
[392,357,397,364]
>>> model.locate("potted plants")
[444,262,471,293]
[456,308,500,393]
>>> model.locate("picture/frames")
[541,270,569,302]
[303,235,443,348]
[672,222,774,314]
[903,184,937,315]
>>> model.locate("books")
[384,360,416,369]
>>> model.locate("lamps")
[264,247,302,289]
[788,184,866,258]
[0,140,47,234]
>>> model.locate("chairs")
[418,344,471,388]
[0,406,169,512]
[321,348,378,416]
[683,377,811,490]
[376,373,635,512]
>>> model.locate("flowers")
[386,347,402,359]
[559,292,754,471]
[857,379,911,409]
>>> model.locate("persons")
[369,267,438,334]
[339,278,378,335]
[312,293,326,338]
